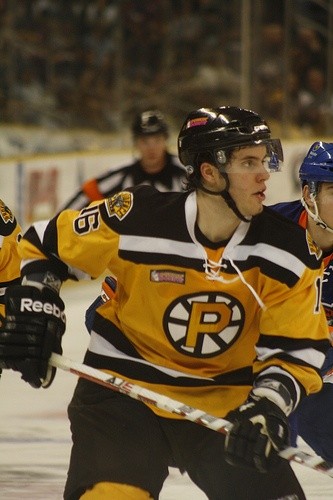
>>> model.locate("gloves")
[222,374,298,473]
[0,282,66,389]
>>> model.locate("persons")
[260,141,333,470]
[0,197,21,323]
[0,104,333,500]
[131,109,181,176]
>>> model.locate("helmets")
[299,141,333,183]
[178,105,271,166]
[133,111,166,135]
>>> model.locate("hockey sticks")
[49,351,333,479]
